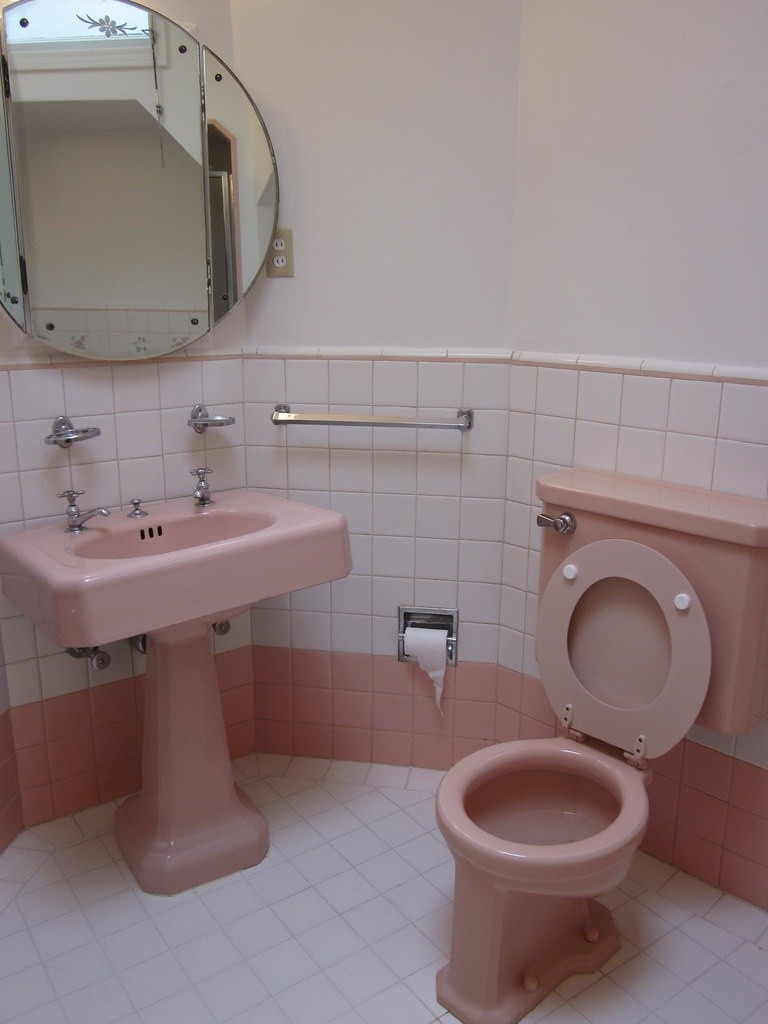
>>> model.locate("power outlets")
[266,228,294,277]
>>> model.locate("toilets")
[435,469,768,1024]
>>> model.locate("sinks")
[0,493,352,646]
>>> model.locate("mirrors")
[1,1,281,362]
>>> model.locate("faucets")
[188,467,212,505]
[56,491,110,536]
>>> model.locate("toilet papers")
[404,627,453,715]
